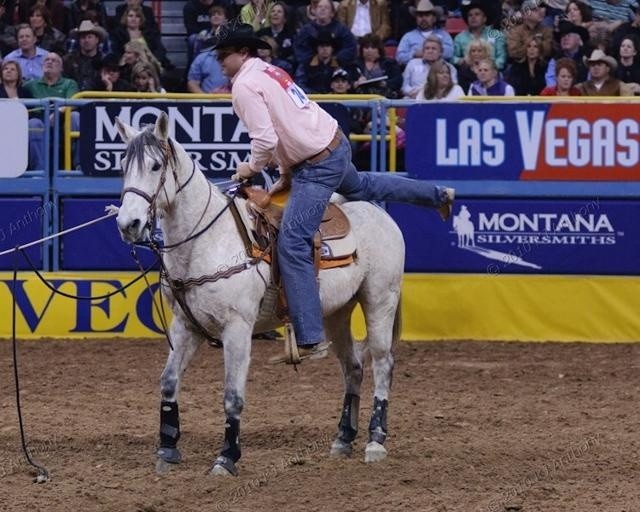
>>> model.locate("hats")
[68,19,106,39]
[198,23,273,54]
[459,2,495,27]
[552,19,591,44]
[90,53,132,74]
[330,68,351,82]
[582,49,619,72]
[408,0,444,19]
[304,30,345,55]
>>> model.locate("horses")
[114,110,406,479]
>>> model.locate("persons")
[196,22,455,366]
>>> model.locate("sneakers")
[435,187,456,224]
[263,338,332,371]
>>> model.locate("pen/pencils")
[357,67,363,76]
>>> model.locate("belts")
[293,125,344,171]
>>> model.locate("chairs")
[373,1,469,121]
